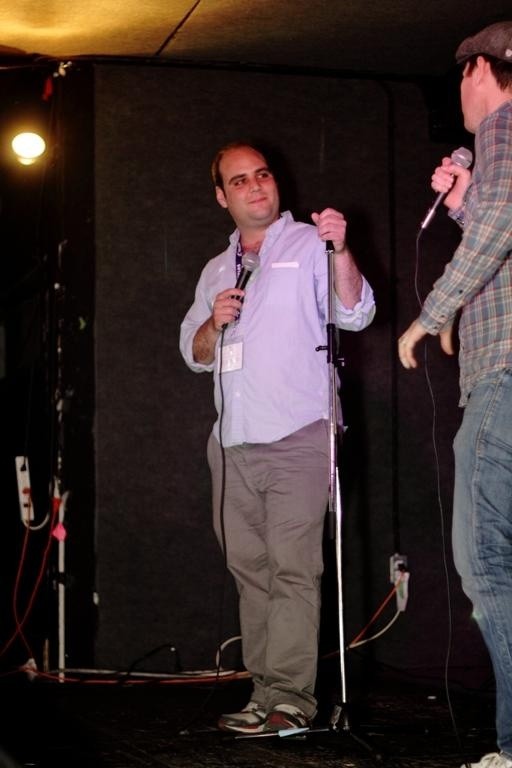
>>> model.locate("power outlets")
[389,554,410,586]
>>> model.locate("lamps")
[11,131,46,164]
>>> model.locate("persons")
[179,143,382,744]
[390,22,512,768]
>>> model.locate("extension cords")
[15,455,35,521]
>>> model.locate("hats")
[454,21,512,64]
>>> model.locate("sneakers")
[217,701,314,740]
[459,752,512,768]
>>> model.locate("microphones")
[420,148,473,229]
[221,252,260,330]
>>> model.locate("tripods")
[220,251,430,768]
